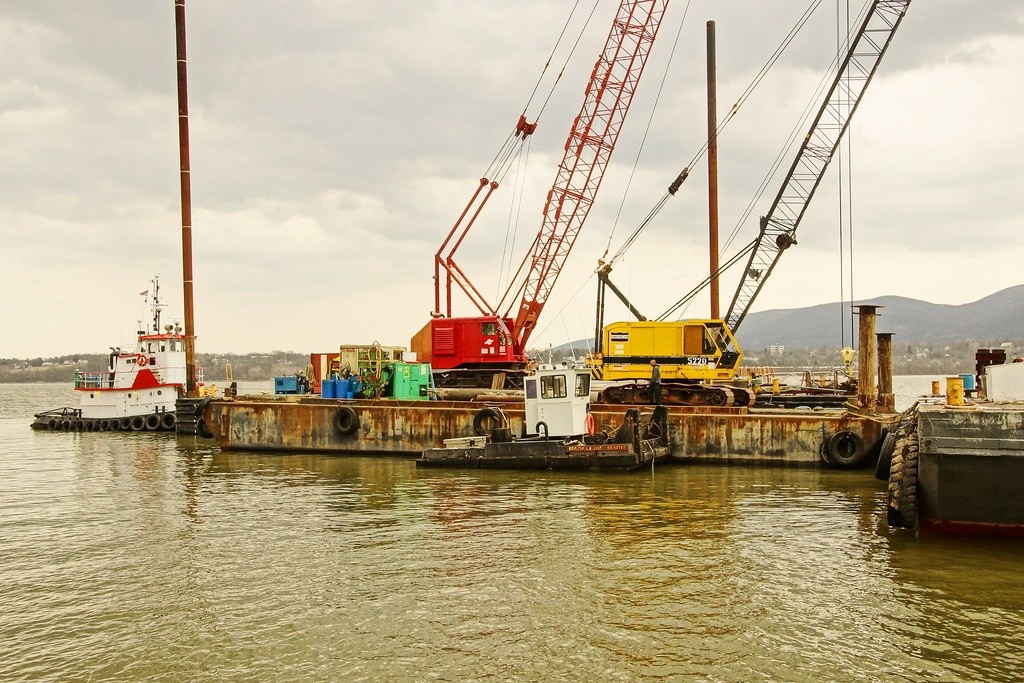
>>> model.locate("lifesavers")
[587,414,594,437]
[136,355,147,367]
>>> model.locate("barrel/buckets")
[334,380,350,399]
[322,379,334,398]
[959,374,974,389]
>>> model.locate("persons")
[648,358,662,405]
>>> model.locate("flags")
[140,290,148,296]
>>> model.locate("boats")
[32,275,184,429]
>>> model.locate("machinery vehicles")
[411,0,671,388]
[594,0,912,409]
